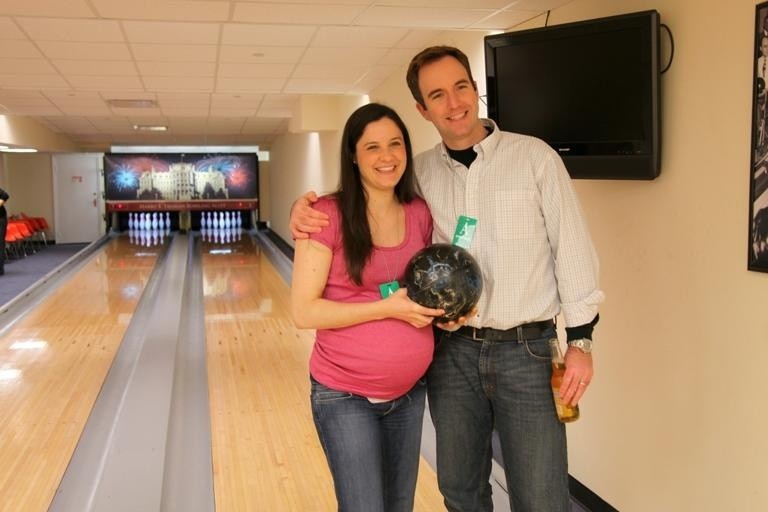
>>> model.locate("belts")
[455,320,557,342]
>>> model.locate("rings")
[580,382,587,387]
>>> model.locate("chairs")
[4,211,50,261]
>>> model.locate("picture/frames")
[747,0,768,274]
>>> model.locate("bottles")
[547,335,583,425]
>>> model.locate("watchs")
[567,337,593,353]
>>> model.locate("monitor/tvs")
[484,8,664,180]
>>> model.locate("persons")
[0,187,10,275]
[284,100,478,511]
[289,44,605,512]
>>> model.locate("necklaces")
[367,207,403,283]
